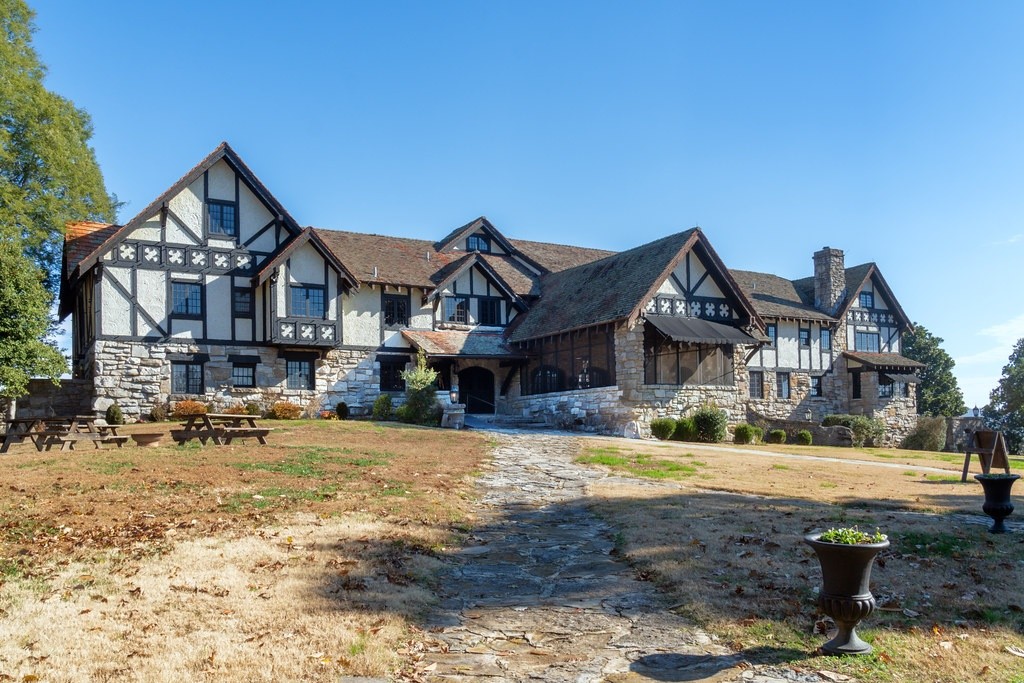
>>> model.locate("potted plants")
[974,472,1022,533]
[803,524,891,658]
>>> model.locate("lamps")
[971,405,980,417]
[804,408,812,422]
[450,385,459,404]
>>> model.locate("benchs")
[1,424,130,444]
[215,427,274,433]
[179,421,248,426]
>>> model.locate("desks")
[46,415,110,450]
[0,418,66,453]
[177,414,269,446]
